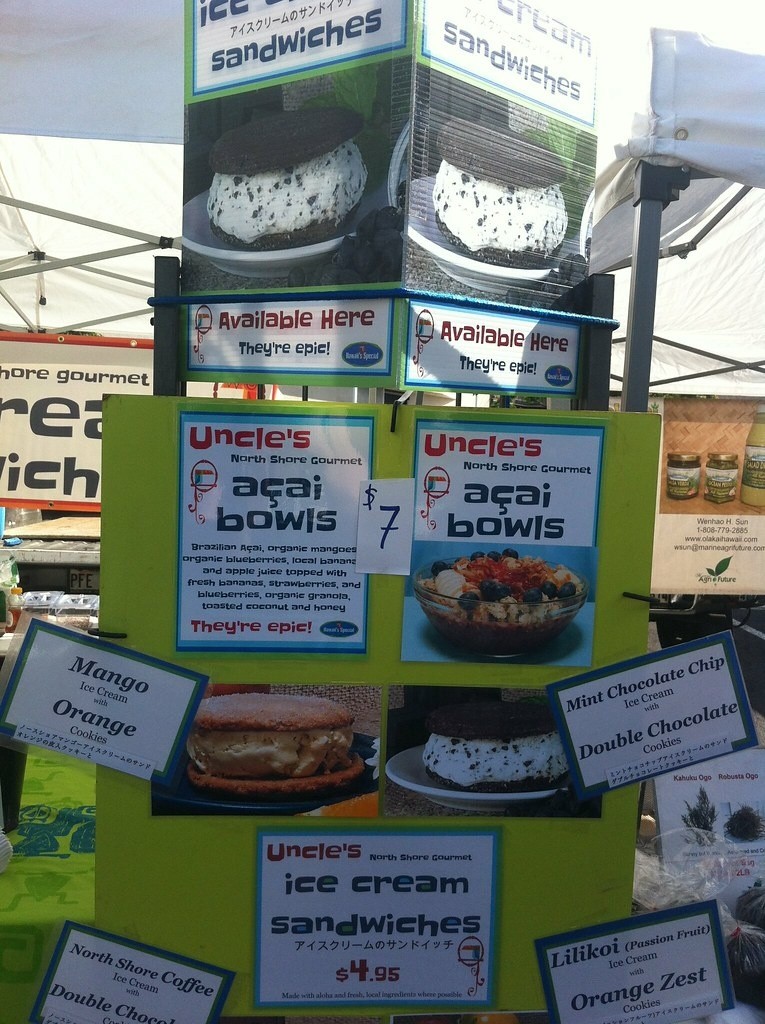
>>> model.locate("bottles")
[740,404,765,506]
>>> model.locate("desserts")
[423,701,571,793]
[186,692,366,795]
[206,108,364,252]
[433,120,566,269]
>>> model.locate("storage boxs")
[18,589,100,631]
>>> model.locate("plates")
[386,745,568,811]
[151,732,379,815]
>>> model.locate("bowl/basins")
[183,189,389,277]
[408,177,580,295]
[413,558,589,656]
